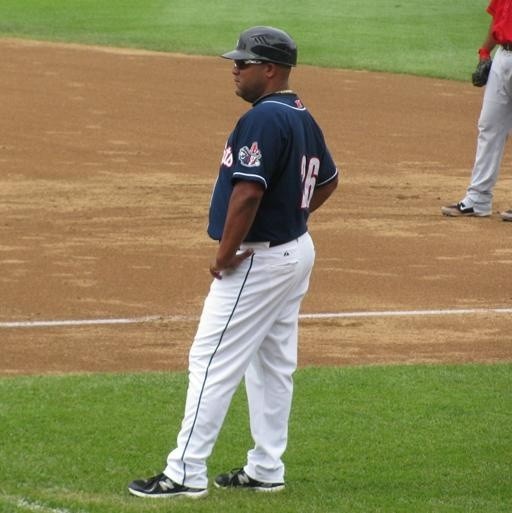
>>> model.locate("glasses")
[233,54,285,68]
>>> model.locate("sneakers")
[499,209,512,221]
[440,200,494,219]
[126,468,211,501]
[211,461,288,495]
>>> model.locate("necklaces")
[251,88,298,106]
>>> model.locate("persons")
[128,25,339,497]
[440,0,512,220]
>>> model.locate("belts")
[501,43,512,52]
[238,241,292,248]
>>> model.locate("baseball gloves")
[472,60,491,86]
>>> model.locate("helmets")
[220,25,299,69]
